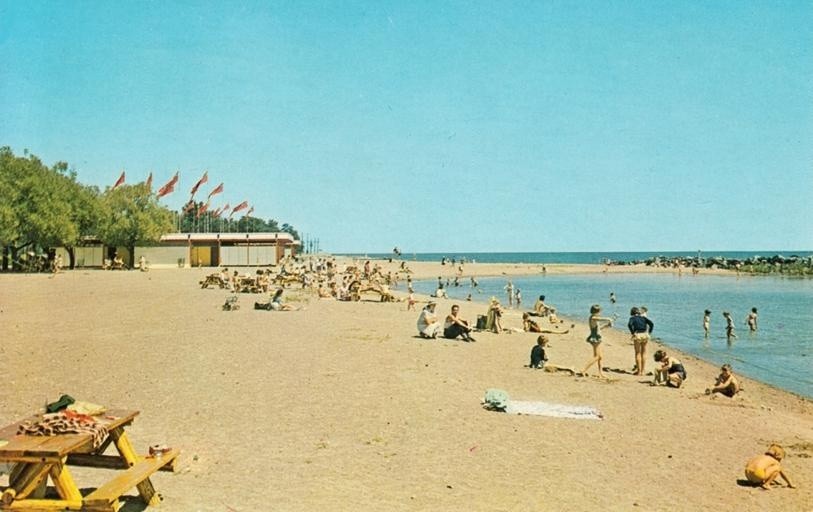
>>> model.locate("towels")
[500,397,608,420]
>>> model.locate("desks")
[0,402,162,510]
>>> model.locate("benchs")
[80,447,179,511]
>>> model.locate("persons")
[628,307,654,377]
[640,307,648,318]
[528,335,550,368]
[750,265,753,274]
[734,261,741,275]
[579,304,613,379]
[703,309,712,338]
[744,443,797,491]
[610,293,615,303]
[654,349,687,388]
[711,364,739,398]
[602,255,699,275]
[744,306,759,332]
[20,251,149,278]
[203,252,416,312]
[421,256,574,343]
[722,311,738,341]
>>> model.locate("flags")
[157,174,179,199]
[111,170,126,191]
[145,172,152,188]
[184,198,255,224]
[209,183,224,196]
[190,172,208,194]
